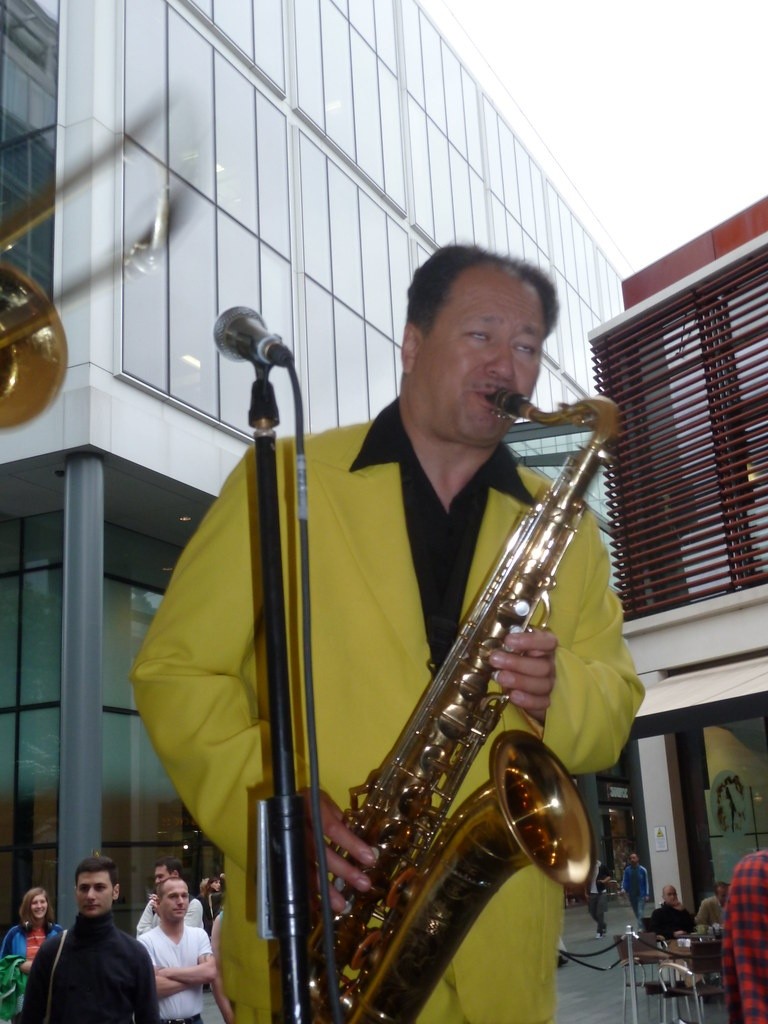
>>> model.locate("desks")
[657,939,722,958]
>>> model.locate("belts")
[163,1014,202,1024]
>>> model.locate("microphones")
[212,306,295,368]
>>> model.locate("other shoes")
[595,933,604,938]
[602,928,607,935]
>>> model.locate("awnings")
[627,654,768,741]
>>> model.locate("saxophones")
[307,386,619,1024]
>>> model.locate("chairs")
[613,918,723,1024]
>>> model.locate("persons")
[128,243,645,1024]
[648,881,729,989]
[720,848,768,1024]
[0,855,226,1024]
[621,852,649,934]
[584,860,612,939]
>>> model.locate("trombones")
[0,88,203,431]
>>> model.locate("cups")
[696,925,706,935]
